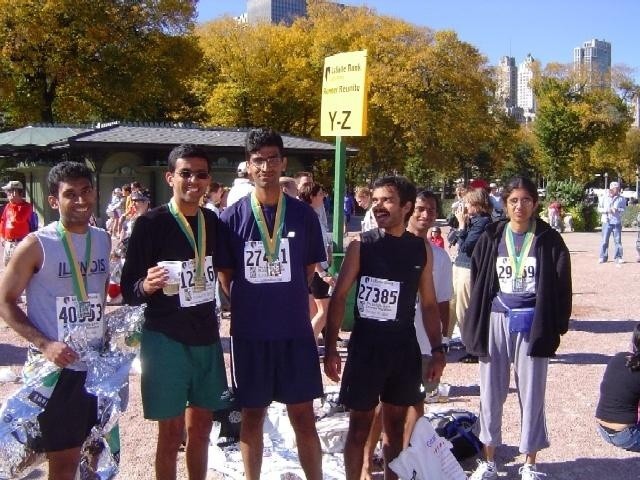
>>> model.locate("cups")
[437,383,452,403]
[156,261,183,296]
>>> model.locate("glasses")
[248,156,283,168]
[170,170,208,181]
[507,198,533,211]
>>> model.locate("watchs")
[431,346,445,354]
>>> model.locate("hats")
[610,182,620,190]
[1,180,23,191]
[238,162,249,177]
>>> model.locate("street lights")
[634,166,640,202]
[445,178,447,186]
[603,172,609,192]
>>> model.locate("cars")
[537,188,546,198]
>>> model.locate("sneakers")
[317,347,325,355]
[461,353,479,363]
[518,464,547,480]
[467,459,498,480]
[336,339,349,346]
[614,257,625,263]
[598,258,604,263]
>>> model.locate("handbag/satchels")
[509,308,534,333]
[426,407,481,461]
[182,385,242,441]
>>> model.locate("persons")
[222,130,325,480]
[1,178,152,306]
[197,158,350,356]
[594,324,640,454]
[1,158,110,480]
[597,181,626,263]
[324,177,445,480]
[462,176,571,480]
[344,179,564,356]
[120,141,220,480]
[361,191,457,480]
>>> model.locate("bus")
[432,186,456,200]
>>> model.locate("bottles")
[328,272,339,297]
